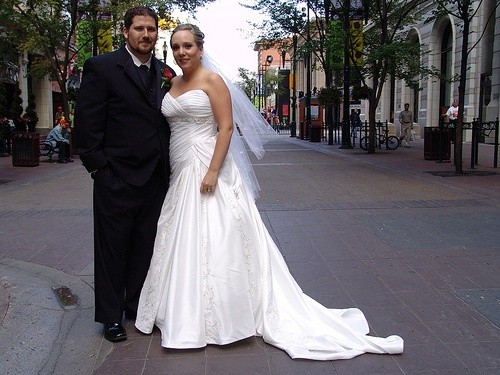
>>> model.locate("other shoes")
[405,142,410,148]
[398,138,402,146]
[57,159,66,163]
[66,158,74,162]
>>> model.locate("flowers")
[160,67,173,89]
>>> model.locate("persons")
[398,103,413,148]
[350,109,361,138]
[275,116,280,134]
[134,24,405,361]
[445,98,458,144]
[74,6,178,342]
[0,104,75,164]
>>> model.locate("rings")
[208,190,211,192]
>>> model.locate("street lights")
[281,35,307,137]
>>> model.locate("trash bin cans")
[11,132,41,166]
[309,125,321,142]
[423,126,452,160]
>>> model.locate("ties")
[139,65,150,89]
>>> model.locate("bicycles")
[360,127,400,151]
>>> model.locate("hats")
[59,120,69,126]
[57,107,62,110]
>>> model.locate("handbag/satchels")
[410,130,414,141]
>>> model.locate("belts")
[403,122,411,123]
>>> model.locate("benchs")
[40,137,59,163]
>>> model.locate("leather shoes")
[125,309,160,332]
[103,321,128,341]
